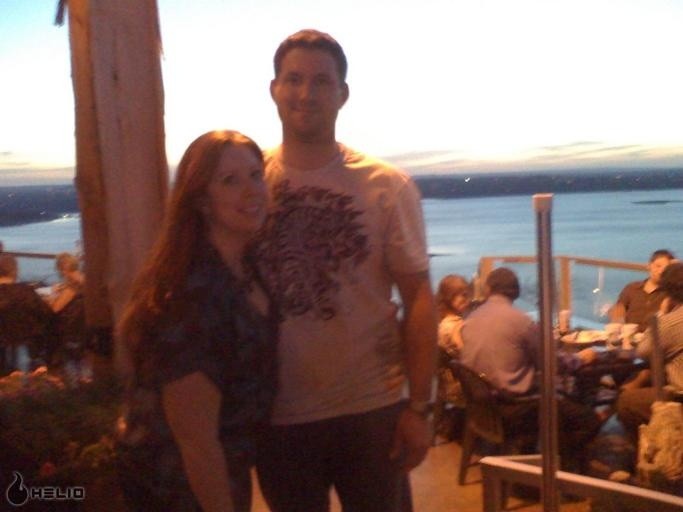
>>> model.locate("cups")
[558,308,570,335]
[607,322,640,352]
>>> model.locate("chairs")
[430,344,554,484]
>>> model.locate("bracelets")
[410,399,435,421]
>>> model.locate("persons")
[0,252,53,378]
[118,129,279,512]
[46,251,83,314]
[606,247,682,390]
[433,273,473,410]
[118,28,435,511]
[607,264,682,484]
[458,265,597,454]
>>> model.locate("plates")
[561,330,609,346]
